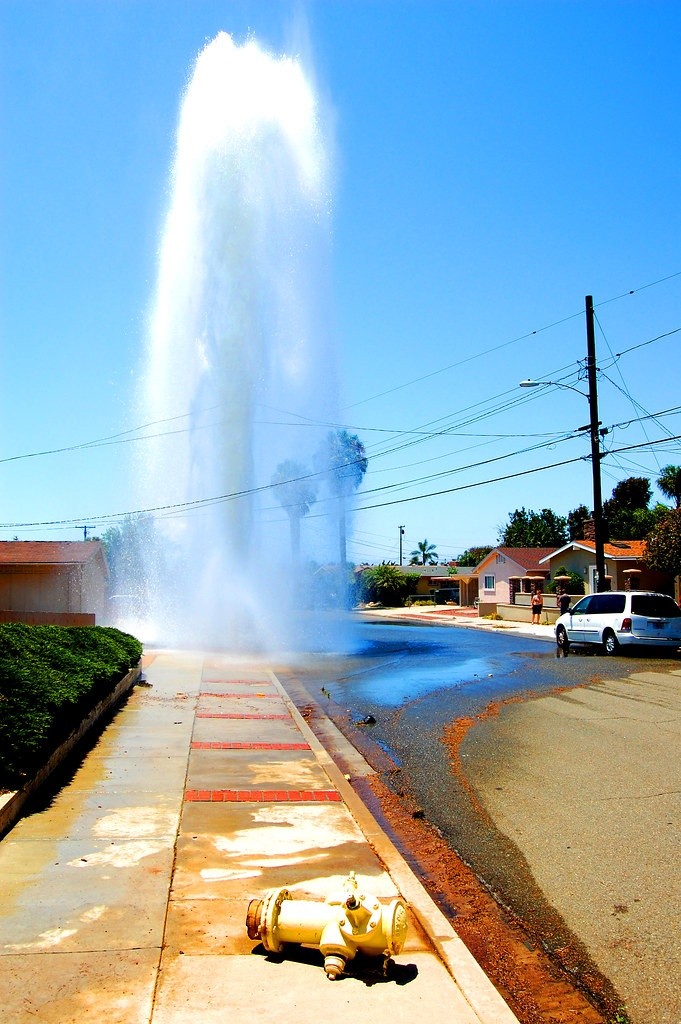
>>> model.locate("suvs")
[554,591,681,656]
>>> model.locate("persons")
[556,589,571,616]
[531,589,543,625]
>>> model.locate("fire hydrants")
[243,870,411,984]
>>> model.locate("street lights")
[518,381,606,593]
